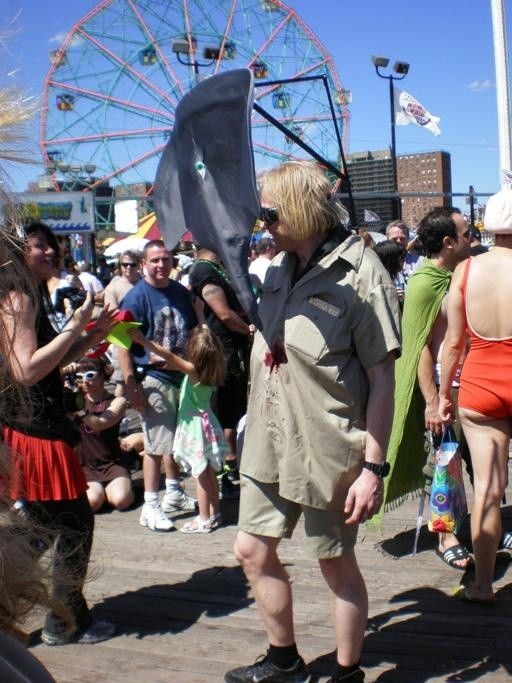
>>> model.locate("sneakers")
[139,503,175,532]
[160,488,199,512]
[216,465,240,501]
[41,619,117,646]
[224,650,312,683]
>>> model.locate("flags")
[393,87,441,140]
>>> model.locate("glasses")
[121,262,137,268]
[259,207,280,227]
[75,369,100,383]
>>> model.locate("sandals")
[179,511,224,534]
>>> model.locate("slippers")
[436,530,512,570]
[451,585,496,606]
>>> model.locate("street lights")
[369,53,412,220]
[171,36,221,88]
[40,161,96,191]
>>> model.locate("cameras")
[53,287,95,314]
